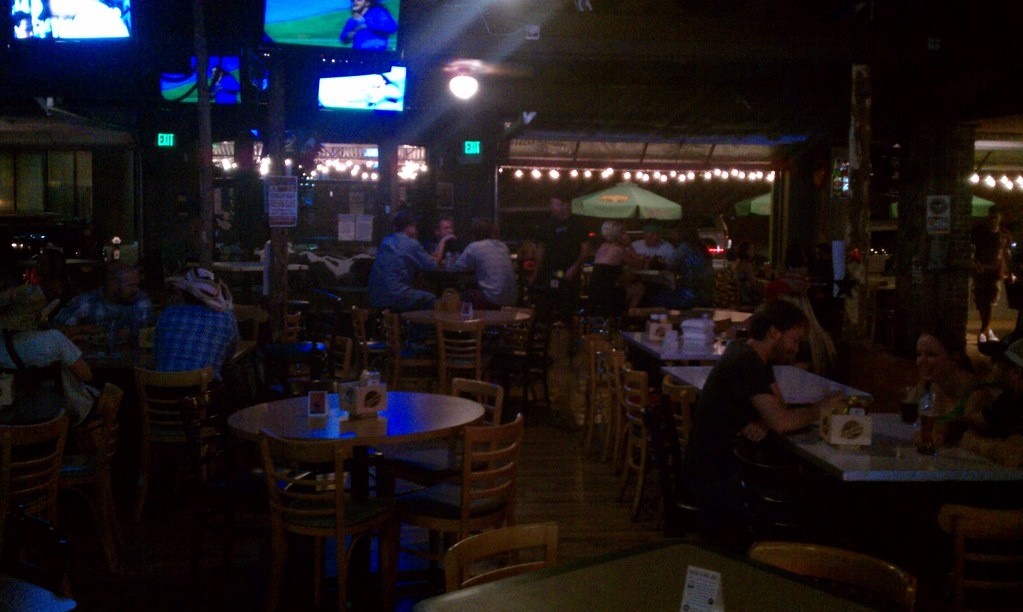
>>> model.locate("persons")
[339,0,398,51]
[0,196,1023,536]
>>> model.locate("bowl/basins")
[681,319,716,355]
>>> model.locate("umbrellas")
[571,180,682,225]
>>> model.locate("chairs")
[0,269,1023,611]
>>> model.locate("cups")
[900,399,919,423]
[919,410,937,448]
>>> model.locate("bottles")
[649,314,669,323]
[444,250,454,272]
[919,381,936,410]
[849,393,872,415]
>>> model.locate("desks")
[412,544,869,610]
[82,337,260,372]
[661,365,871,508]
[619,328,741,368]
[227,390,487,580]
[788,413,1022,578]
[405,305,531,410]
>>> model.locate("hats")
[164,266,232,313]
[0,284,60,330]
